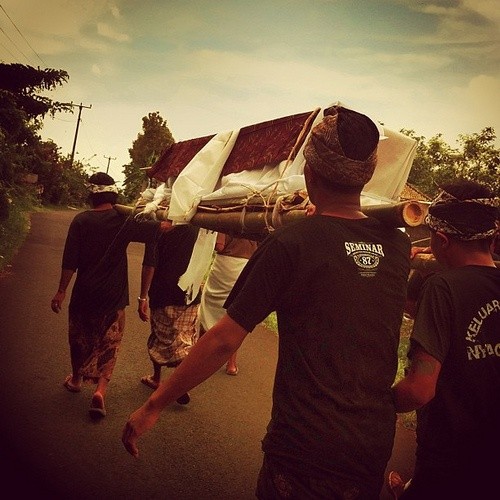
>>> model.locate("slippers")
[141,375,160,389]
[225,363,240,375]
[176,392,190,405]
[63,375,83,392]
[389,471,404,500]
[88,406,106,416]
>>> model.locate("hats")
[303,105,379,192]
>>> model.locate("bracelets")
[137,296,148,302]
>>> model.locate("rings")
[55,304,58,306]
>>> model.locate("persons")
[121,102,404,499]
[48,166,178,419]
[136,183,203,409]
[195,225,261,377]
[390,159,500,499]
